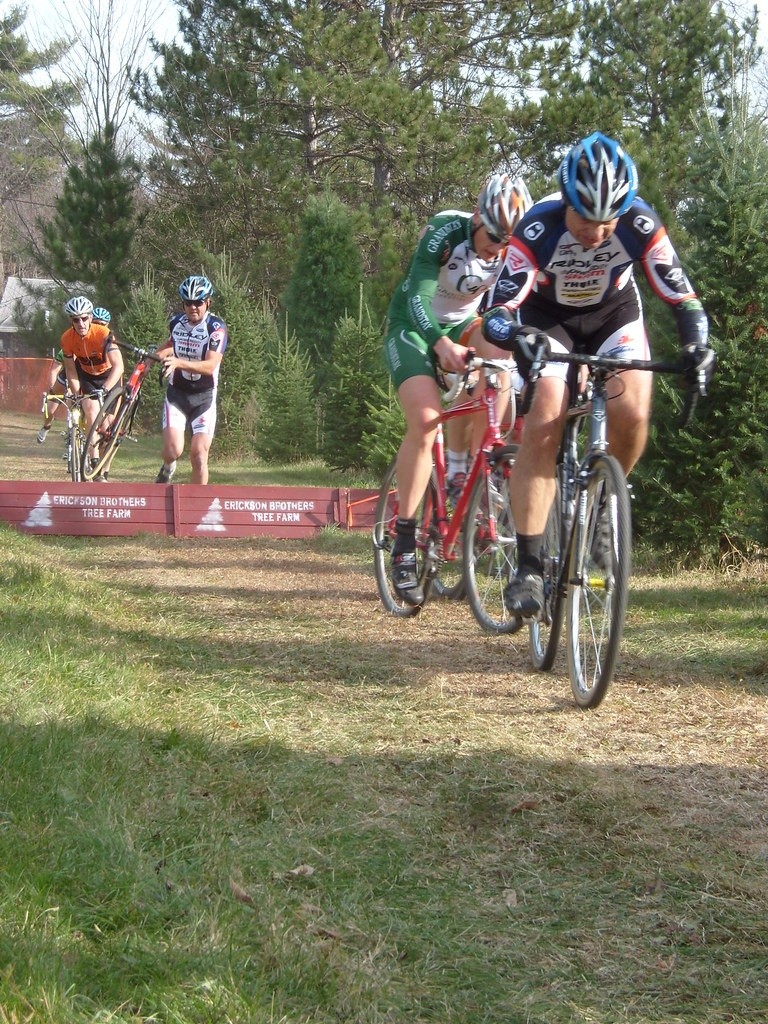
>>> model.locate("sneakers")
[390,547,424,604]
[92,474,108,482]
[37,427,49,444]
[584,506,614,569]
[443,472,467,510]
[155,462,176,484]
[504,565,545,616]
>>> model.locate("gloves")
[510,325,551,382]
[89,386,108,400]
[49,389,57,395]
[678,341,715,392]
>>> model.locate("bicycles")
[79,330,170,481]
[371,348,561,635]
[519,340,706,708]
[42,386,105,483]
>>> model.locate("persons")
[383,173,537,604]
[142,276,229,484]
[36,296,125,483]
[476,131,717,625]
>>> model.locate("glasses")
[72,314,91,323]
[484,231,510,248]
[182,297,208,307]
[93,321,107,327]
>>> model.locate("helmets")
[179,276,213,302]
[65,296,93,317]
[478,173,534,243]
[558,131,638,220]
[92,307,111,323]
